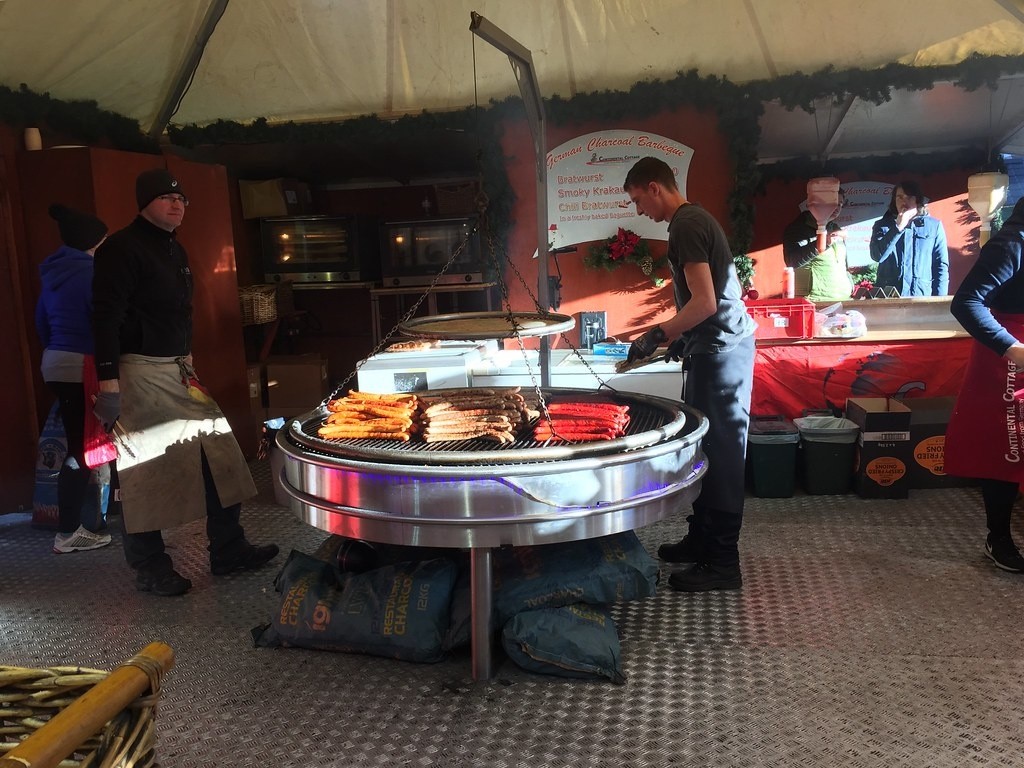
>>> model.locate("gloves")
[92,391,121,434]
[627,324,670,363]
[665,338,685,363]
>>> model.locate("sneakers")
[210,544,279,575]
[984,532,1024,571]
[136,565,192,595]
[669,559,742,591]
[658,535,708,562]
[53,523,112,553]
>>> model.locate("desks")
[369,281,496,347]
[749,320,973,421]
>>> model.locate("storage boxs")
[266,352,330,409]
[593,342,631,356]
[745,297,816,341]
[905,398,980,488]
[843,398,911,500]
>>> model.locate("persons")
[782,187,854,302]
[90,169,279,596]
[950,197,1024,572]
[35,207,111,553]
[623,156,759,591]
[869,180,949,297]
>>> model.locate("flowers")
[847,263,876,297]
[582,226,650,273]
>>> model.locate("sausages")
[317,389,422,442]
[534,396,631,440]
[420,386,540,445]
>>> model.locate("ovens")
[377,214,483,288]
[259,213,361,283]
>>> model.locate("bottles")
[782,267,795,299]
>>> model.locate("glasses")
[155,195,188,206]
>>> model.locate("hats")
[49,203,108,252]
[136,169,187,213]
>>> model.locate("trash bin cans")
[747,414,800,497]
[793,416,861,494]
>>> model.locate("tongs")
[90,392,139,460]
[615,350,667,373]
